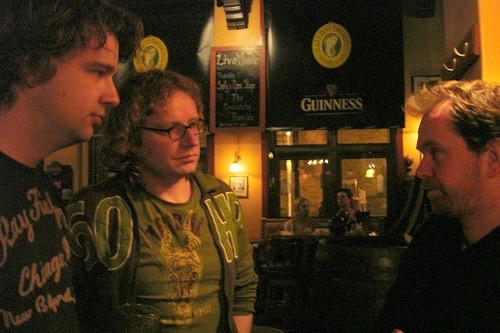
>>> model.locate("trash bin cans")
[252,236,315,332]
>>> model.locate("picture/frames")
[209,45,266,135]
[229,176,248,198]
[411,74,440,93]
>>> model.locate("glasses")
[138,120,206,142]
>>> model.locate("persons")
[0,0,143,333]
[373,80,500,333]
[63,69,260,333]
[283,188,375,280]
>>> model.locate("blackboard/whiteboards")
[209,46,267,134]
